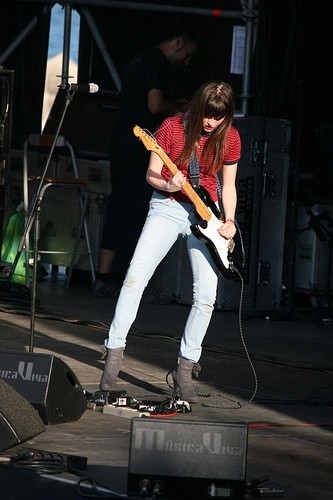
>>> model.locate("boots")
[100,347,124,391]
[172,356,201,403]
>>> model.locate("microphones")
[58,82,99,93]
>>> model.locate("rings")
[181,182,186,185]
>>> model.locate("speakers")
[0,377,45,453]
[0,349,88,424]
[126,417,249,500]
[35,90,119,161]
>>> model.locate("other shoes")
[142,284,172,304]
[90,278,121,296]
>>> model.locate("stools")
[23,133,95,285]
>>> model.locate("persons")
[100,81,241,403]
[88,28,198,305]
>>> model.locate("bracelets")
[226,219,235,223]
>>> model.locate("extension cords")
[102,404,150,419]
[16,446,88,470]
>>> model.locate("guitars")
[134,124,235,275]
